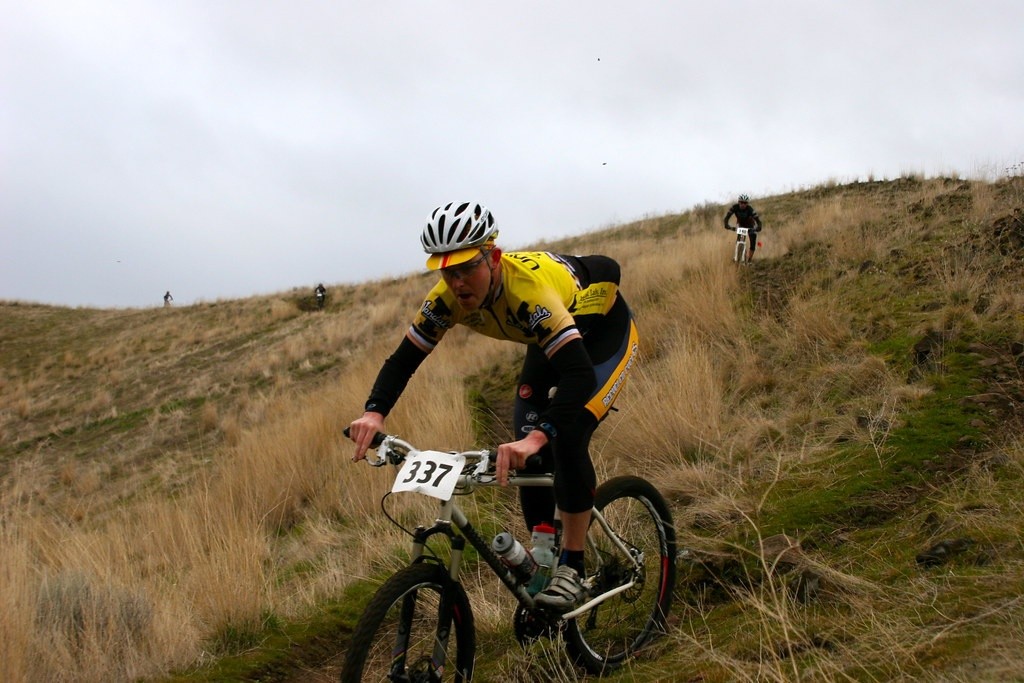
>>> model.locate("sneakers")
[534,564,593,609]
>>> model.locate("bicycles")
[340,423,687,683]
[724,225,758,271]
[312,291,325,310]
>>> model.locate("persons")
[348,201,640,610]
[163,291,173,302]
[723,194,762,263]
[314,283,326,305]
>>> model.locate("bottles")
[525,522,556,595]
[492,532,539,579]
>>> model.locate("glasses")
[435,245,497,281]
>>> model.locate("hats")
[426,238,495,270]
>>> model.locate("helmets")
[421,200,500,253]
[738,194,750,204]
